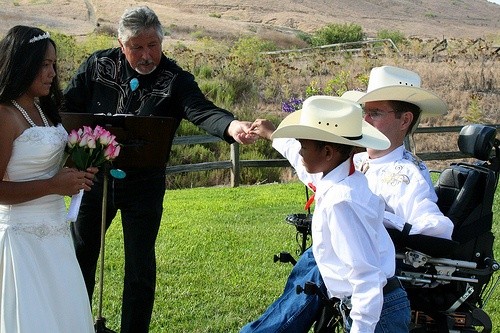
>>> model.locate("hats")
[340,65,447,118]
[270,95,391,151]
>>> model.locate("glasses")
[362,107,412,122]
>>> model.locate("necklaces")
[10,100,49,127]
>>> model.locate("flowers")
[64,126,125,222]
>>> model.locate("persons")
[0,25,99,333]
[249,95,411,333]
[238,66,448,333]
[61,5,258,333]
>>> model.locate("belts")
[382,277,401,296]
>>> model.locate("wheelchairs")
[273,124,500,333]
